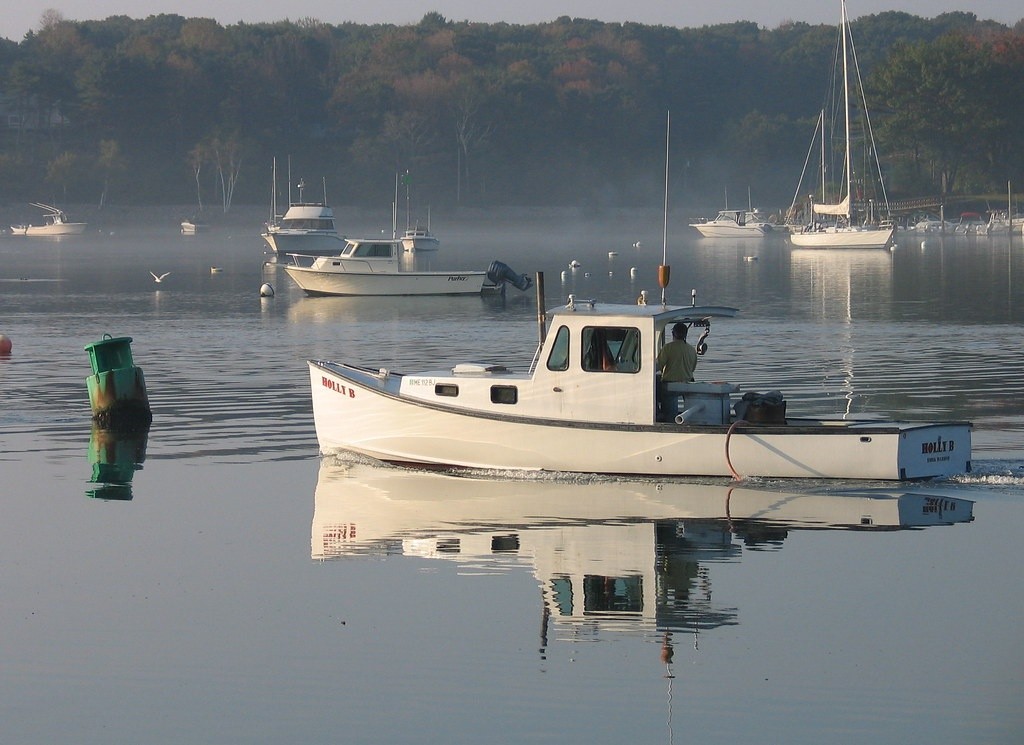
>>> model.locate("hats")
[671,322,688,339]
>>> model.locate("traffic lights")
[955,212,987,236]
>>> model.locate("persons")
[655,323,697,382]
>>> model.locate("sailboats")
[785,0,898,248]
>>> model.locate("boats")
[311,454,978,737]
[915,220,956,235]
[401,183,440,251]
[10,201,87,237]
[309,109,976,481]
[261,167,348,254]
[986,209,1024,235]
[181,215,211,234]
[687,186,791,236]
[284,173,533,297]
